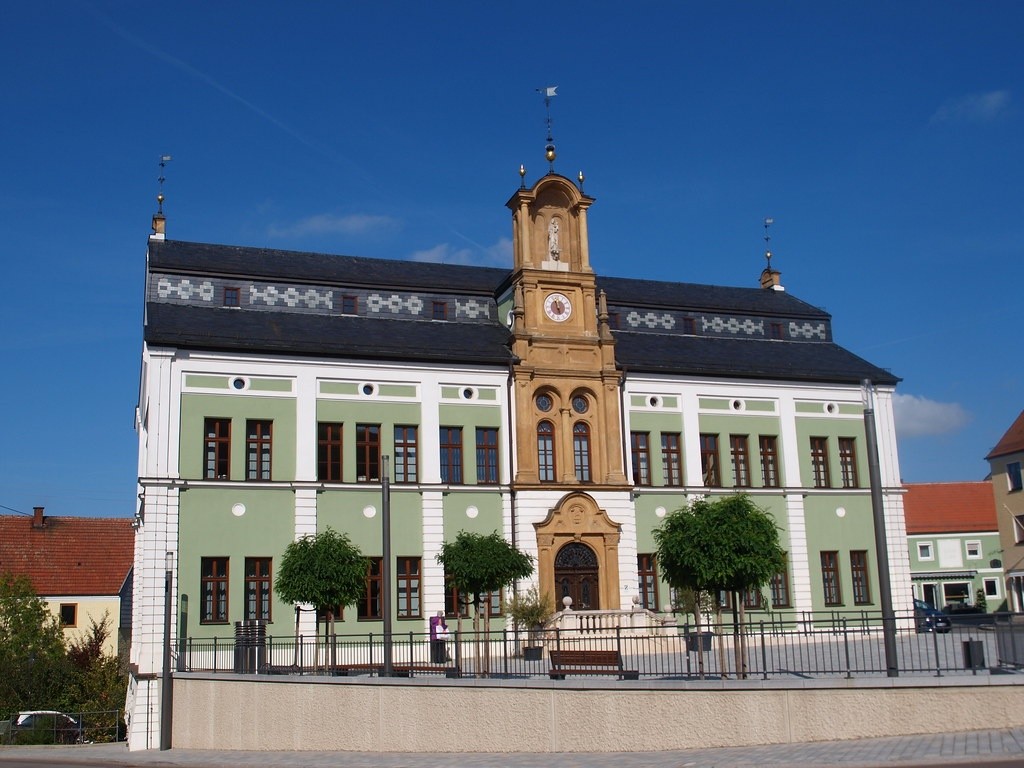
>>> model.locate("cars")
[914,598,956,633]
[16,711,77,727]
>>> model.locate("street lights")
[160,551,173,751]
[859,378,898,678]
[381,454,393,678]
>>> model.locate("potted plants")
[503,584,557,661]
[674,590,716,649]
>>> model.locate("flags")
[546,86,559,97]
[162,156,171,161]
[766,218,773,223]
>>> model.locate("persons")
[431,611,449,664]
[548,219,559,254]
[960,590,968,608]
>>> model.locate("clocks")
[544,292,572,322]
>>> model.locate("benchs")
[548,649,623,681]
